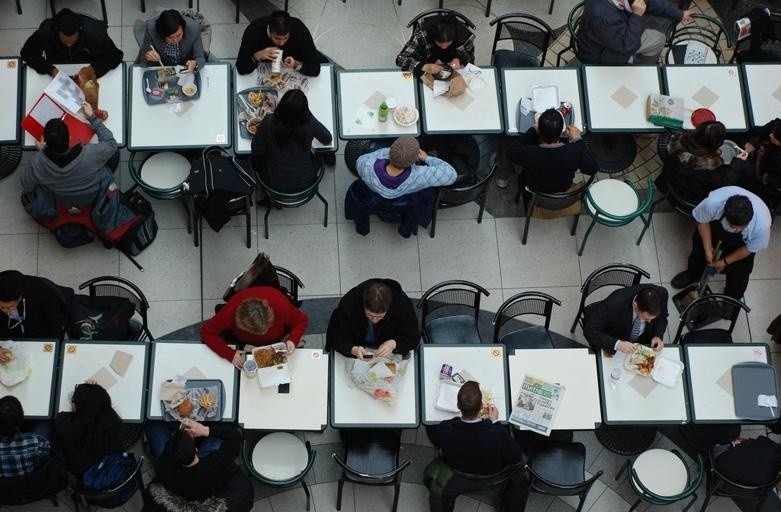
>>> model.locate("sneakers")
[489,153,511,188]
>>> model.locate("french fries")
[200,396,212,407]
[248,89,263,105]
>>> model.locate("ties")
[628,317,642,342]
[364,320,375,346]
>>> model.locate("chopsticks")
[150,45,166,69]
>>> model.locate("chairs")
[68,456,147,511]
[2,275,153,341]
[331,437,412,512]
[615,447,706,511]
[526,441,603,512]
[2,454,63,509]
[492,292,562,348]
[676,296,752,345]
[705,439,781,512]
[243,433,318,511]
[437,444,528,507]
[222,264,306,348]
[0,1,781,257]
[570,263,651,334]
[416,279,491,343]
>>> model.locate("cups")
[386,97,397,109]
[271,50,283,75]
[241,360,258,379]
[610,368,623,380]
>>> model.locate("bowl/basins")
[246,118,262,134]
[182,83,197,96]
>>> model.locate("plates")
[435,65,455,81]
[393,105,419,126]
[466,76,488,94]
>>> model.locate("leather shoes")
[721,300,736,321]
[671,270,699,290]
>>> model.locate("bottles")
[377,101,389,123]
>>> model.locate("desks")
[2,339,779,454]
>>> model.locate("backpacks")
[98,191,158,272]
[82,451,137,508]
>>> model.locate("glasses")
[366,314,383,319]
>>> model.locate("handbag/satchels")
[181,145,257,202]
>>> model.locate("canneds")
[560,103,571,118]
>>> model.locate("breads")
[178,401,193,416]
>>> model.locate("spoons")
[145,78,152,93]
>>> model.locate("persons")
[200,285,311,372]
[47,378,141,484]
[355,135,459,201]
[506,109,602,196]
[574,0,698,66]
[581,282,670,354]
[20,101,119,210]
[135,7,222,75]
[744,117,781,229]
[235,10,331,79]
[395,13,477,76]
[19,7,126,86]
[140,415,244,503]
[1,269,71,340]
[0,394,52,479]
[433,378,525,475]
[670,185,774,321]
[667,119,754,201]
[250,87,334,212]
[324,276,422,360]
